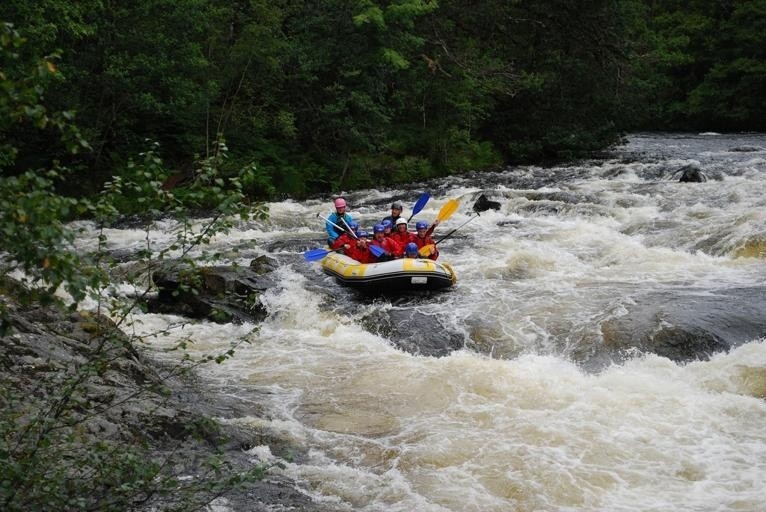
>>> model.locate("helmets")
[405,242,418,254]
[381,220,393,229]
[416,221,427,229]
[348,221,358,232]
[374,223,385,235]
[390,202,402,213]
[335,198,347,209]
[395,217,408,231]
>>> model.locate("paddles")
[303,246,345,261]
[316,212,385,258]
[407,193,430,223]
[432,199,460,229]
[417,211,481,256]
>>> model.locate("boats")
[319,246,457,293]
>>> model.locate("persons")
[323,197,440,264]
